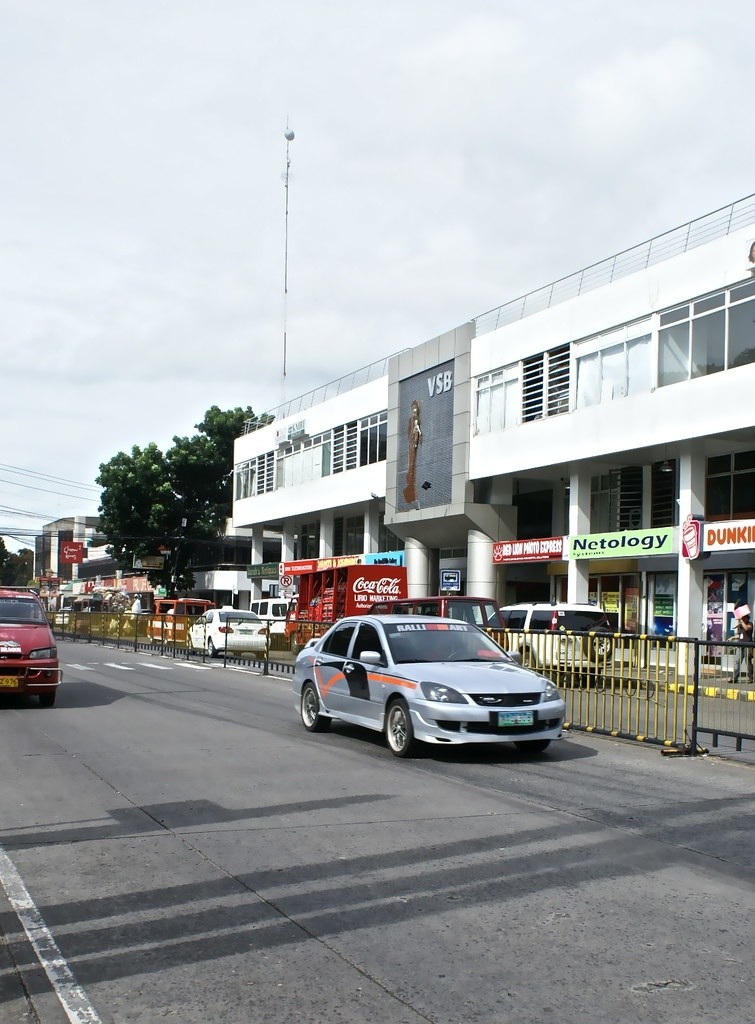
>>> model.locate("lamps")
[560,463,571,489]
[658,446,674,472]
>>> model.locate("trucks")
[285,565,408,655]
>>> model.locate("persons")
[130,593,141,637]
[167,605,174,614]
[727,615,754,684]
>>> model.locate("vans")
[250,599,298,650]
[488,604,612,688]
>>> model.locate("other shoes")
[727,680,737,683]
[747,680,753,683]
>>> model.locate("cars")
[0,586,63,706]
[367,596,509,651]
[186,606,271,660]
[291,614,565,758]
